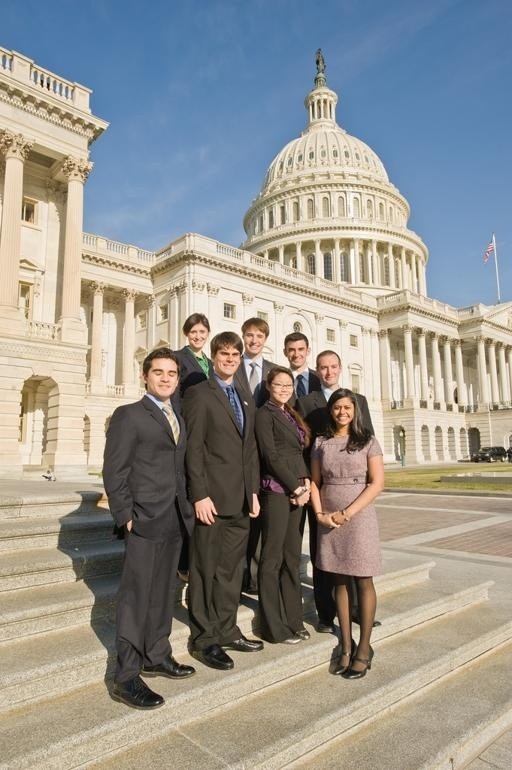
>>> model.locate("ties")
[163,404,182,446]
[296,375,306,399]
[247,363,260,395]
[225,385,243,432]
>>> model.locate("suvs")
[470,446,507,463]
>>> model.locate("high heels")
[343,643,375,678]
[334,639,356,675]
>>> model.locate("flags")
[483,239,493,263]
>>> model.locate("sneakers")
[139,651,195,679]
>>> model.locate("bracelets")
[342,510,350,521]
[297,488,307,497]
[315,512,325,517]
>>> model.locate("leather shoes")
[317,614,336,633]
[290,625,309,640]
[267,629,301,645]
[219,630,263,652]
[188,641,234,671]
[350,606,381,628]
[110,674,165,710]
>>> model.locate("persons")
[236,318,279,595]
[310,389,384,680]
[101,347,196,711]
[183,332,263,670]
[255,366,311,644]
[174,313,213,583]
[295,350,381,634]
[283,333,321,400]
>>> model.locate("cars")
[505,447,512,462]
